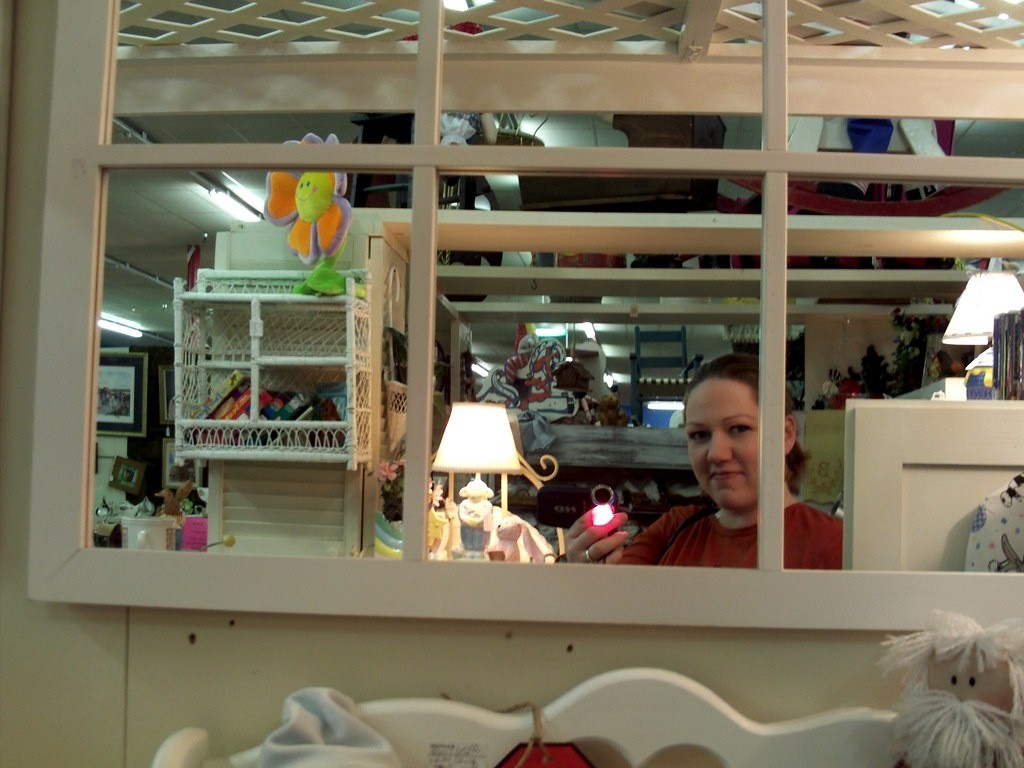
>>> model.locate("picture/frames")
[158,364,175,425]
[96,350,148,437]
[162,438,203,490]
[108,455,145,495]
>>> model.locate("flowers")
[374,437,405,523]
[885,297,954,396]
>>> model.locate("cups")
[120,516,177,551]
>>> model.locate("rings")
[586,551,592,560]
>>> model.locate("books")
[185,369,314,446]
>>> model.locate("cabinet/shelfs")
[172,266,377,474]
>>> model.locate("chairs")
[629,325,703,427]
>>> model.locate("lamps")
[941,273,1023,390]
[431,402,523,517]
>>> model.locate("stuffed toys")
[496,516,544,564]
[262,133,364,295]
[965,472,1024,572]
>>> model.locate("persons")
[879,612,1024,768]
[565,353,844,568]
